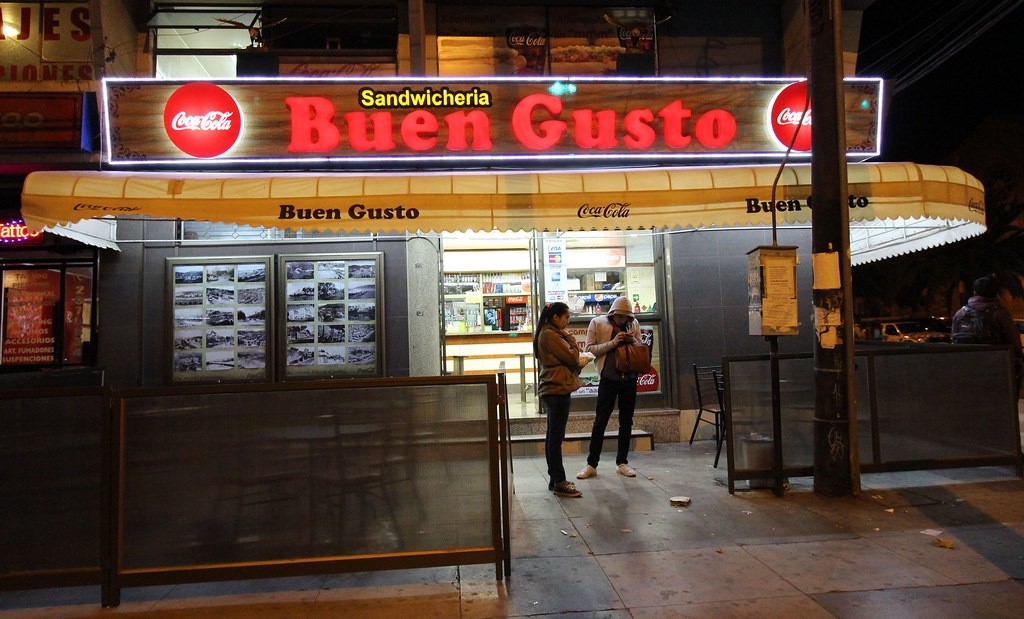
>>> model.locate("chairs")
[688,363,724,445]
[12,365,106,529]
[310,405,408,551]
[202,395,305,545]
[712,368,774,467]
[347,390,430,520]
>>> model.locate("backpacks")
[951,305,1003,344]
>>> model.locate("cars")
[871,317,1024,356]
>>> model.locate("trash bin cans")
[740,436,776,488]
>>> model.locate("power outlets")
[631,270,640,286]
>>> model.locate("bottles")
[635,303,639,313]
[510,308,526,331]
[632,300,638,313]
[595,303,601,313]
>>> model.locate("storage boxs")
[504,296,528,304]
[580,275,604,290]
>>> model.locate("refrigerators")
[505,296,526,332]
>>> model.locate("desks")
[128,403,224,532]
[732,383,813,462]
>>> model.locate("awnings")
[21,161,987,267]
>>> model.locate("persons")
[533,301,593,498]
[576,296,643,479]
[952,280,1024,401]
[855,323,865,339]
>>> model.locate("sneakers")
[616,463,636,477]
[577,464,597,479]
[549,480,576,491]
[553,483,582,497]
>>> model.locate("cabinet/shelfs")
[440,265,628,332]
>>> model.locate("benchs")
[442,352,537,402]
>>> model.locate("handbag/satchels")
[615,339,651,374]
